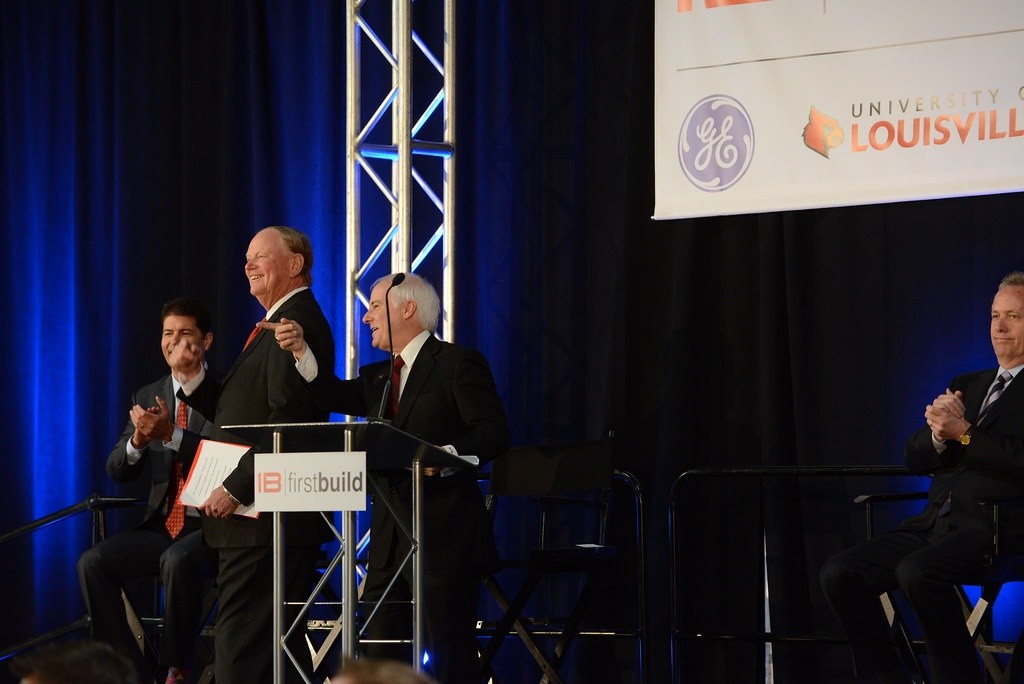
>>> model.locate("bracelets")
[222,485,240,505]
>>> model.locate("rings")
[141,423,144,428]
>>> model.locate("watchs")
[959,423,978,446]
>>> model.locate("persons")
[255,274,512,684]
[324,660,431,684]
[9,643,141,684]
[167,224,335,684]
[79,297,219,684]
[818,272,1024,684]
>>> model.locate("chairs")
[474,428,616,684]
[853,491,1024,684]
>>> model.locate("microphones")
[368,272,406,425]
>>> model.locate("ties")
[937,370,1012,521]
[165,402,188,538]
[243,318,268,353]
[388,355,405,423]
